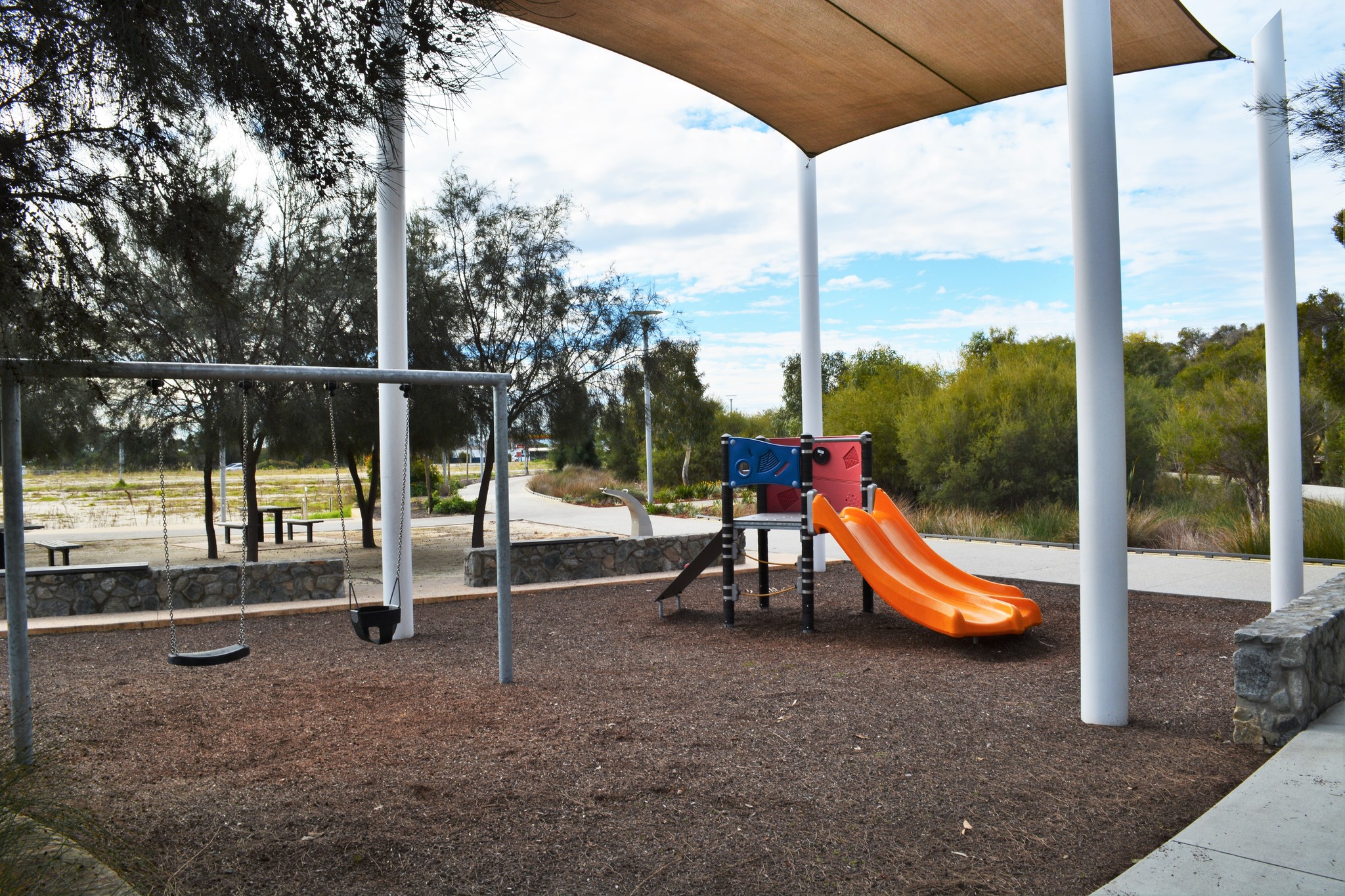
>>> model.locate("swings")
[155,394,248,666]
[327,392,414,645]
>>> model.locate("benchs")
[34,540,83,567]
[214,521,248,544]
[282,518,324,542]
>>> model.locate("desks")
[0,521,45,570]
[236,505,301,544]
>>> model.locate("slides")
[812,487,1043,636]
